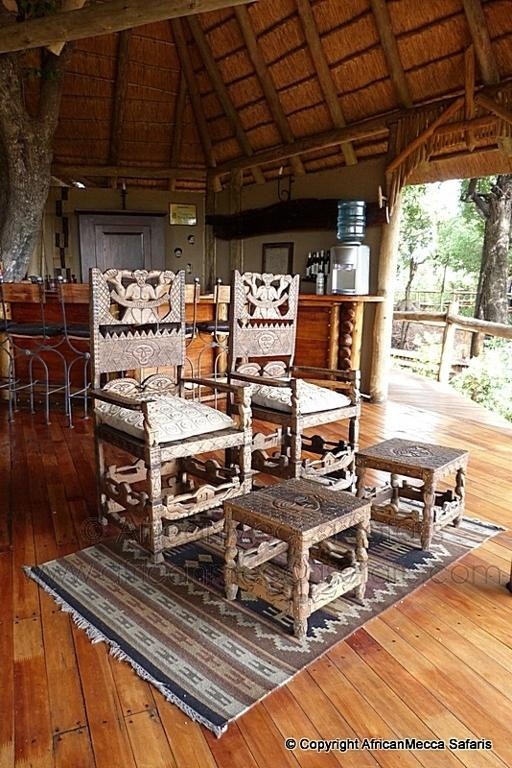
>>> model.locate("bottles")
[315,273,325,295]
[305,248,332,279]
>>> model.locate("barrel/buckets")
[337,200,367,242]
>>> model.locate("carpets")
[22,474,510,740]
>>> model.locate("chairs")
[90,268,361,566]
[0,274,256,430]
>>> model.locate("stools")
[222,437,471,641]
